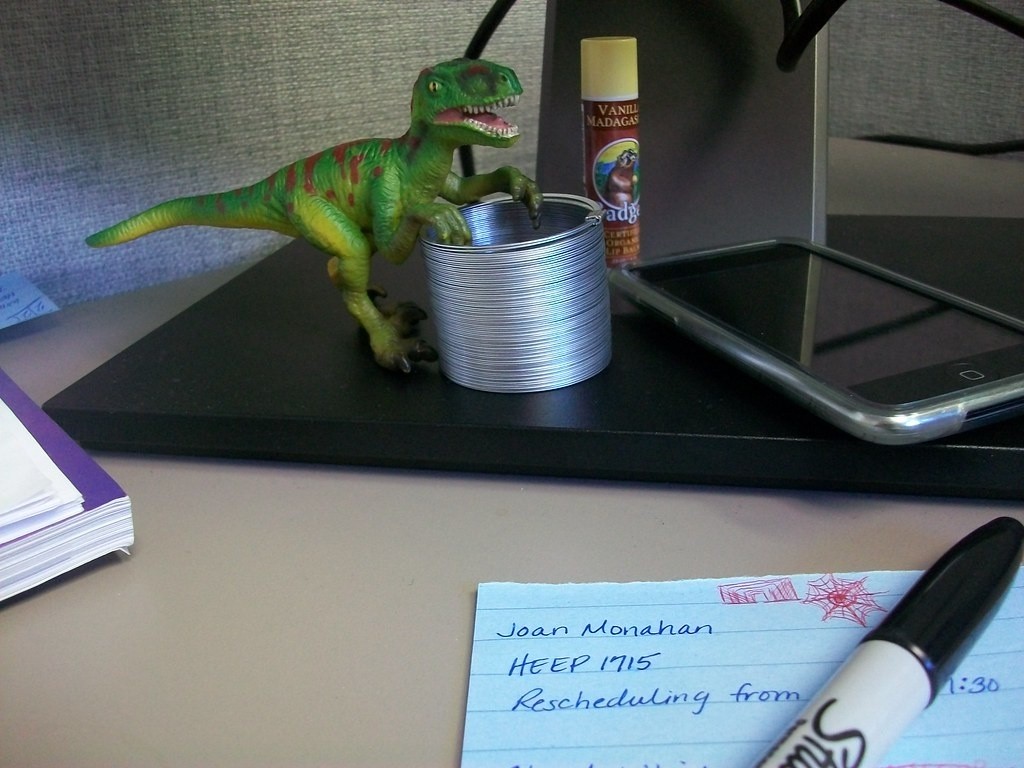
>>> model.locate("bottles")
[580,37,640,264]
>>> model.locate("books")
[0,366,135,610]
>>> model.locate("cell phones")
[611,236,1024,445]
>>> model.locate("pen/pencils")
[758,513,1023,767]
